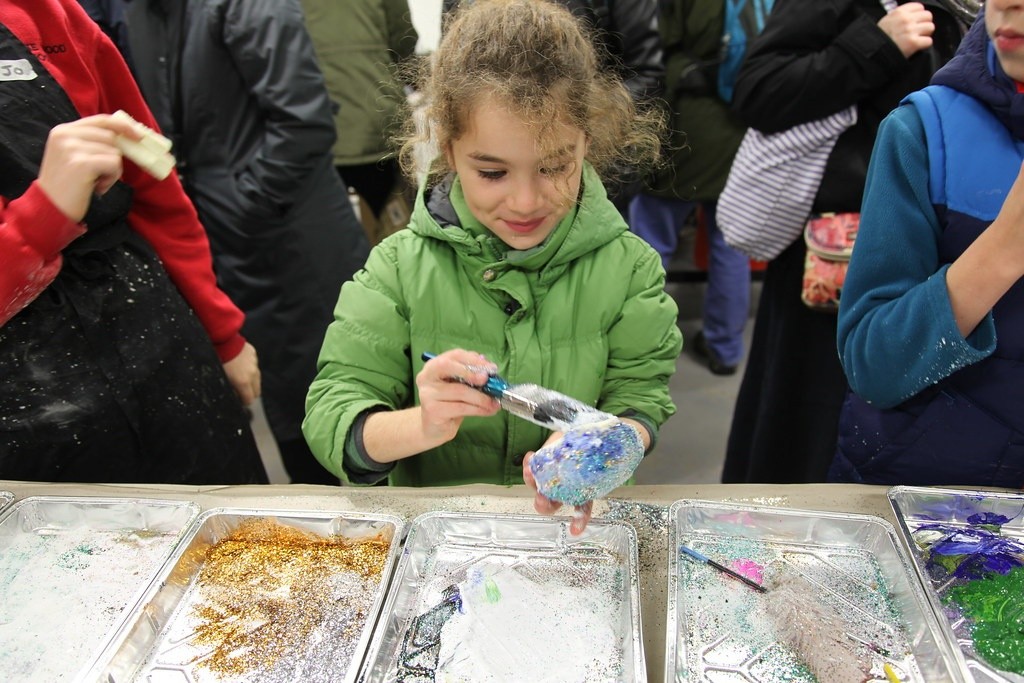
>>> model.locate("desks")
[0,478,1024,683]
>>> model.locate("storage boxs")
[664,498,966,683]
[886,483,1024,683]
[354,508,650,683]
[0,494,202,682]
[84,506,403,682]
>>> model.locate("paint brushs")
[682,547,767,594]
[420,350,577,426]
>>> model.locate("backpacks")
[700,1,780,108]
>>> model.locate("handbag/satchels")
[711,2,902,266]
[804,204,869,312]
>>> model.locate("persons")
[78,0,367,488]
[0,0,269,485]
[300,0,684,537]
[837,2,1023,489]
[625,0,776,376]
[440,0,663,212]
[720,0,983,486]
[296,0,419,246]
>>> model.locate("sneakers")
[688,329,740,378]
